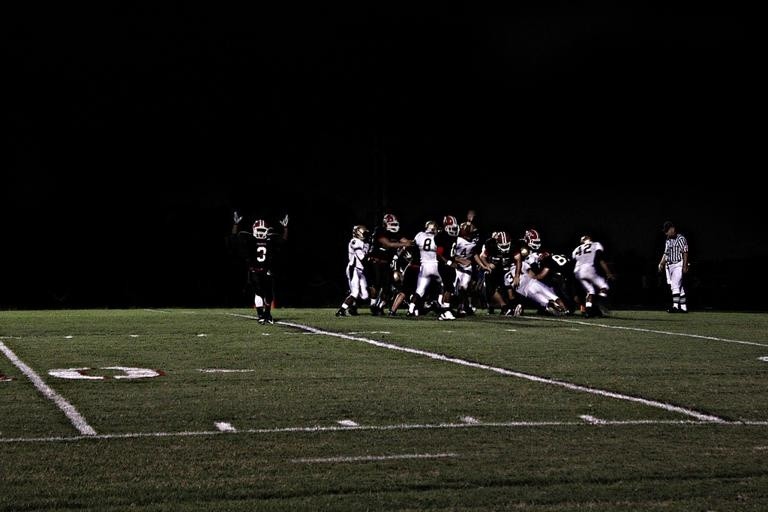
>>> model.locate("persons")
[230,210,291,324]
[658,220,691,313]
[334,208,614,320]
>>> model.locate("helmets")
[580,236,590,244]
[252,220,271,240]
[425,216,542,255]
[352,213,399,242]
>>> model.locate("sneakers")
[258,314,274,325]
[668,307,688,313]
[336,290,609,320]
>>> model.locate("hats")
[662,221,674,232]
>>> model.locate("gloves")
[279,214,289,227]
[233,212,243,225]
[658,264,663,272]
